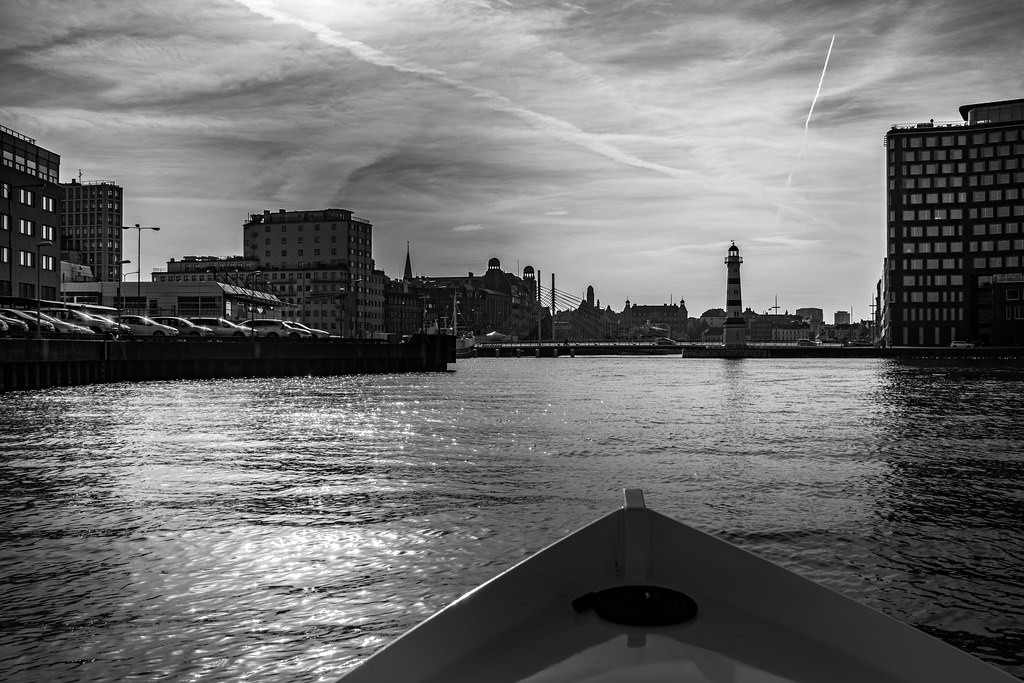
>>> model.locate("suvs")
[949,341,976,349]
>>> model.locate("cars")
[285,321,329,339]
[283,321,312,339]
[111,315,180,337]
[187,317,259,338]
[149,316,214,337]
[847,338,874,346]
[0,306,131,337]
[238,319,286,338]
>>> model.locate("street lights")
[2,182,47,316]
[117,260,131,341]
[33,240,53,340]
[249,270,260,338]
[121,223,160,325]
[353,279,363,343]
[338,288,345,339]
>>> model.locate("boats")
[655,337,676,345]
[796,337,823,347]
[406,289,475,359]
